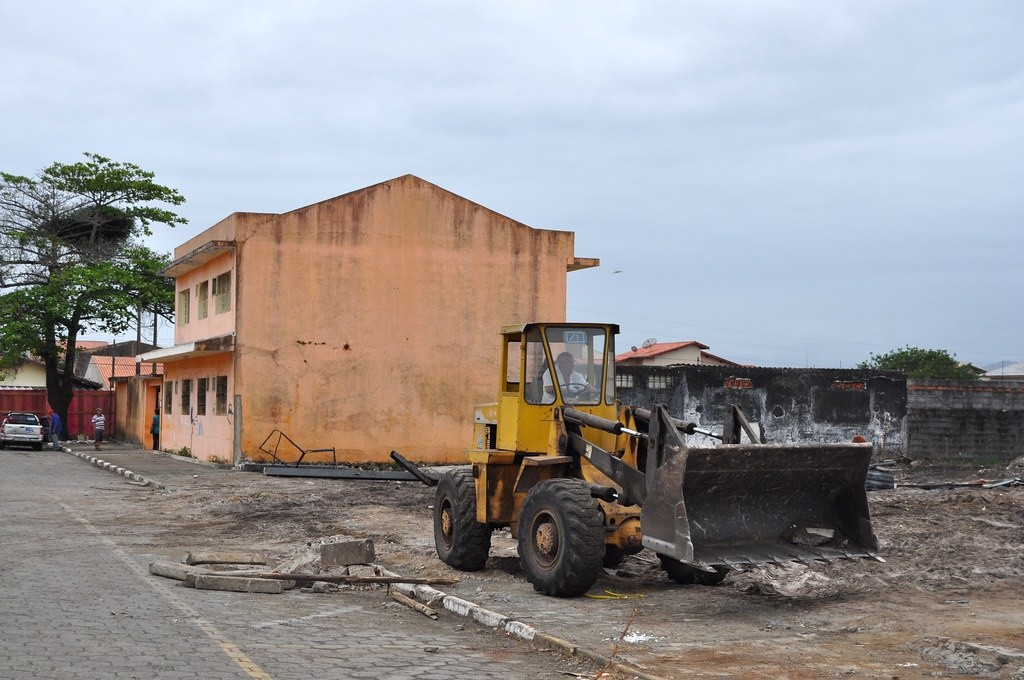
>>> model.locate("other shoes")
[50,447,61,451]
[95,447,102,451]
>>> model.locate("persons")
[47,409,62,451]
[540,351,599,404]
[149,408,159,450]
[92,408,106,451]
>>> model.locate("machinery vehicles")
[389,322,886,599]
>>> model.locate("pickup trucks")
[0,412,44,451]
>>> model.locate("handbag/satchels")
[150,425,155,434]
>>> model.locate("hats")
[558,352,578,364]
[47,409,54,414]
